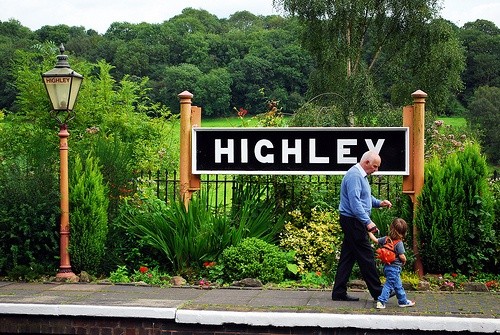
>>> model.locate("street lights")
[39,43,85,282]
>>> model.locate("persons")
[331,150,397,301]
[367,218,416,309]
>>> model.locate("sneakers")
[376,302,385,308]
[399,300,415,308]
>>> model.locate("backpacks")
[376,236,401,265]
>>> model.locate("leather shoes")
[376,289,396,300]
[332,294,359,301]
